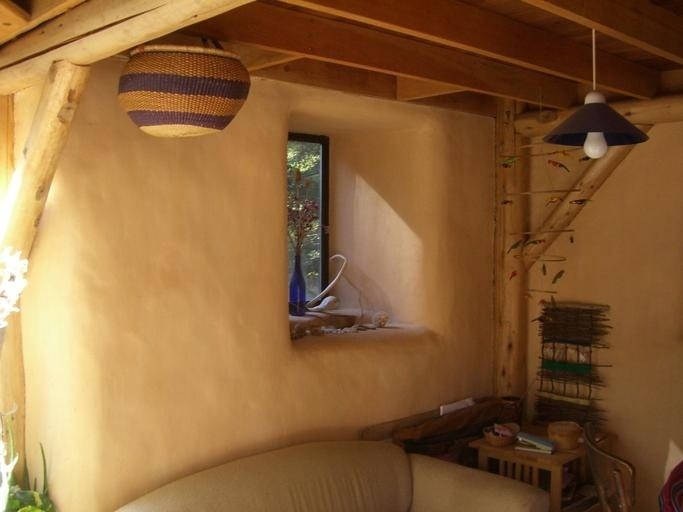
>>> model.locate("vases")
[289,256,307,317]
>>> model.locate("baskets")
[118,32,252,137]
[483,423,520,446]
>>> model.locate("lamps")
[541,26,651,161]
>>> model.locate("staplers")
[515,431,556,455]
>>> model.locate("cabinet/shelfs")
[466,423,593,512]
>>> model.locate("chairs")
[580,427,639,511]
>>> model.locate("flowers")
[285,167,333,255]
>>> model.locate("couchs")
[107,436,549,512]
